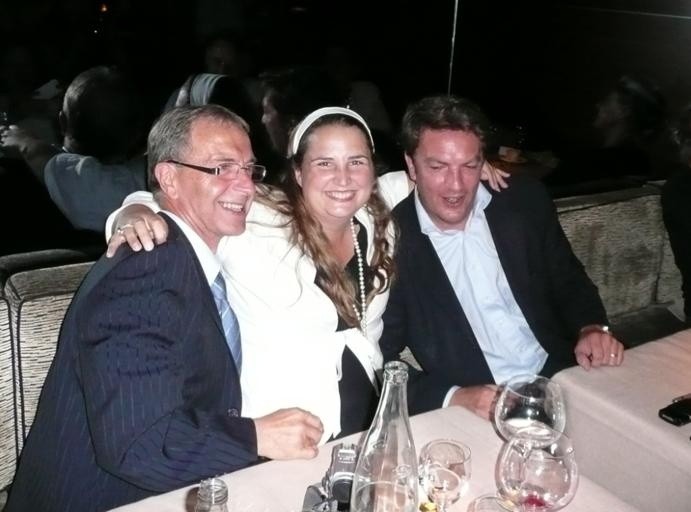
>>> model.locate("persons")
[379,95,624,422]
[2,107,323,512]
[661,102,691,317]
[544,72,670,187]
[0,28,353,250]
[105,106,511,445]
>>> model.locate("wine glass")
[495,375,566,496]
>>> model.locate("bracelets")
[582,325,613,334]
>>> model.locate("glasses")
[165,158,268,183]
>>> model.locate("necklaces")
[349,224,368,339]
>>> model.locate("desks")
[551,326,691,471]
[107,385,691,512]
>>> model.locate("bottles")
[189,478,230,512]
[322,361,422,512]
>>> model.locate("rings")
[609,353,619,357]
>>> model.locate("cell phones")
[659,397,691,425]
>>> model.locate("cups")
[420,427,580,512]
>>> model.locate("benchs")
[1,180,685,490]
